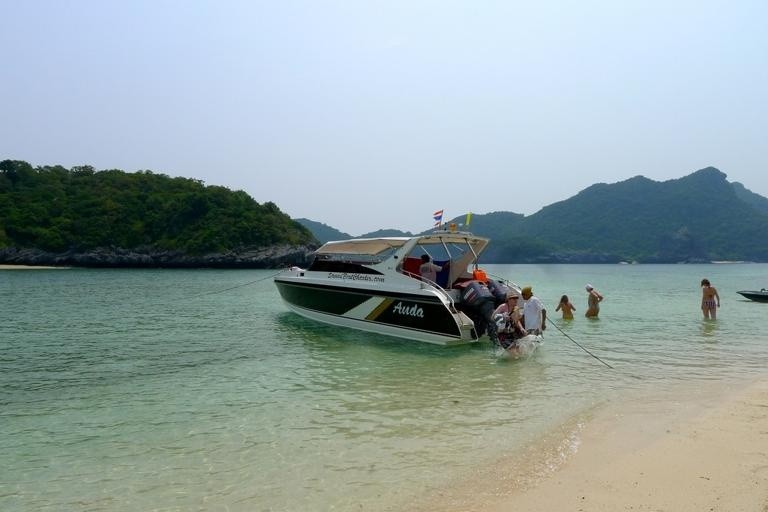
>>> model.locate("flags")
[465,211,472,225]
[433,210,442,227]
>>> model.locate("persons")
[555,295,576,320]
[417,253,451,289]
[584,284,604,321]
[516,284,547,345]
[700,278,721,322]
[489,291,530,360]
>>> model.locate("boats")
[272,230,528,346]
[735,288,768,303]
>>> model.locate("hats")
[507,291,519,299]
